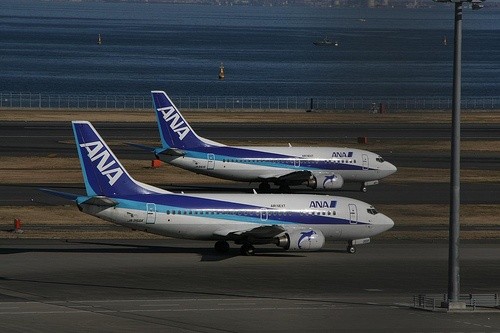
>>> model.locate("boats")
[313,34,341,47]
[98,32,103,48]
[219,61,225,80]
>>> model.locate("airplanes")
[71,119,396,259]
[147,90,397,194]
